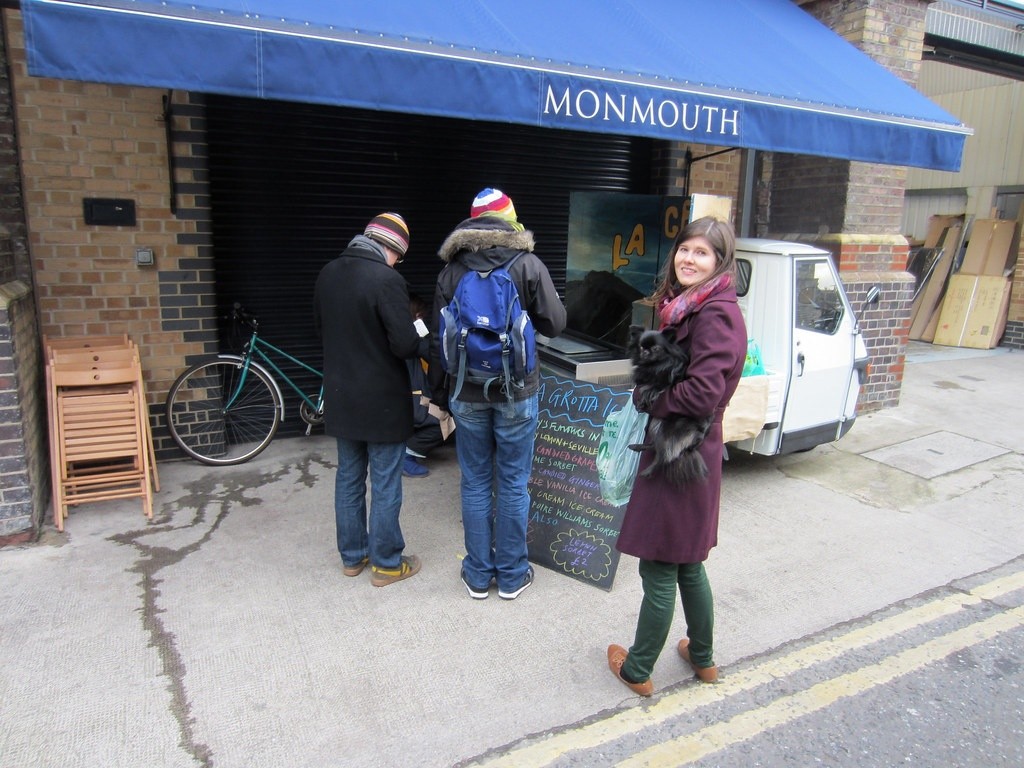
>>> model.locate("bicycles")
[165,313,323,466]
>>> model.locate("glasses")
[396,251,404,263]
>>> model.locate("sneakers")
[499,565,534,598]
[345,555,369,576]
[370,555,421,587]
[679,640,718,683]
[461,567,489,599]
[608,644,653,696]
[400,452,429,478]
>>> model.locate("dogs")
[626,324,709,483]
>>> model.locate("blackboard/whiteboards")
[529,366,648,595]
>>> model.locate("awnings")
[20,0,974,172]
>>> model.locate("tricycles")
[535,237,881,456]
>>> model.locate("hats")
[471,188,516,222]
[364,212,410,256]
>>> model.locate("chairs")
[41,334,159,533]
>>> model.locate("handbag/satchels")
[595,385,649,508]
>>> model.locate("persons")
[428,187,568,600]
[314,213,422,587]
[606,218,749,696]
[401,301,453,477]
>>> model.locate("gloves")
[632,382,653,407]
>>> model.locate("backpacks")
[438,251,537,387]
